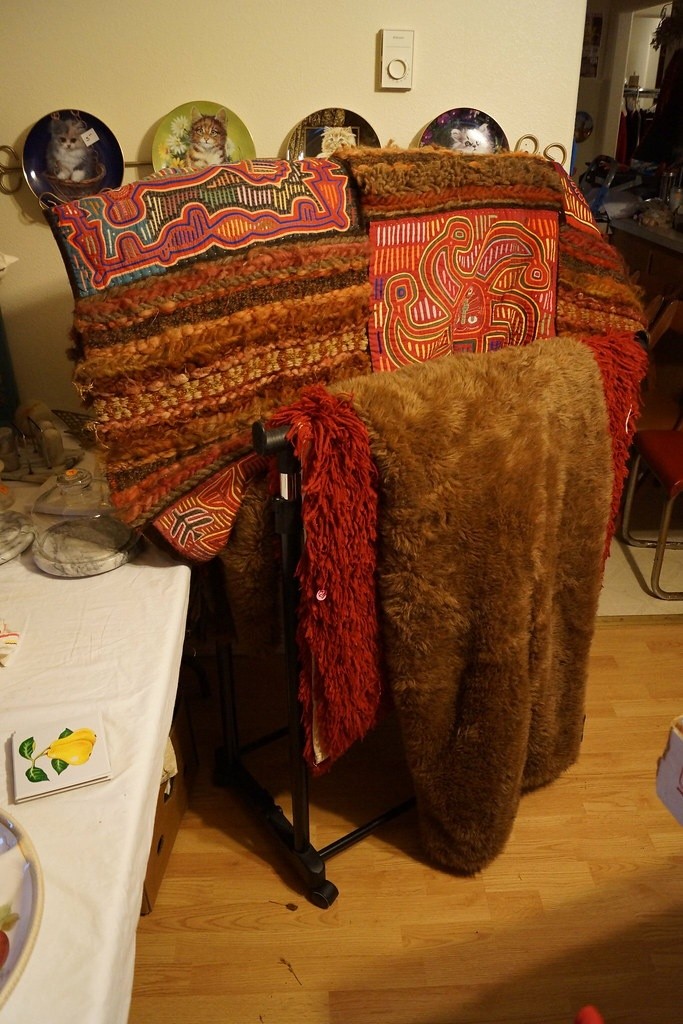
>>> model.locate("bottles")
[30,467,132,563]
[660,164,683,233]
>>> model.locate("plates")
[22,109,124,208]
[574,112,593,143]
[151,102,256,173]
[286,108,382,159]
[417,108,509,156]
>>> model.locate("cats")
[46,110,96,183]
[180,106,230,168]
[449,123,493,151]
[316,125,357,159]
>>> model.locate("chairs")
[615,294,683,600]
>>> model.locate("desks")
[0,474,206,1024]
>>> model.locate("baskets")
[52,409,97,436]
